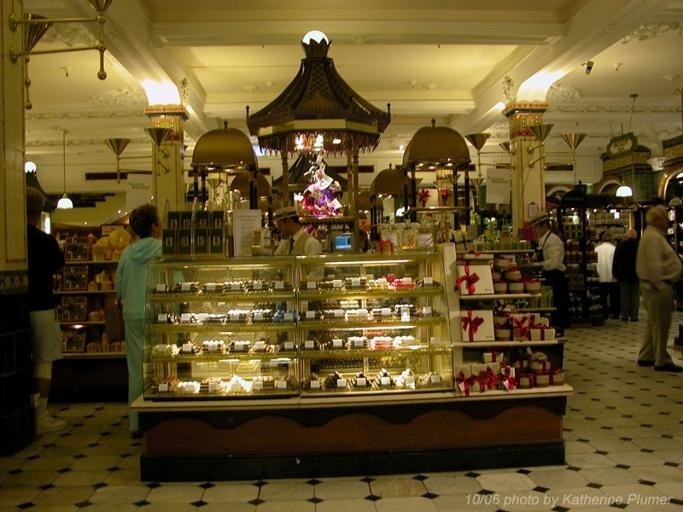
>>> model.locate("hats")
[270,207,301,223]
[528,212,550,228]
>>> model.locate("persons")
[527,210,570,337]
[115,203,164,440]
[637,205,683,373]
[272,208,324,373]
[587,229,641,321]
[26,186,67,434]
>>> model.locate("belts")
[640,278,674,286]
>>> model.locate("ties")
[288,237,295,256]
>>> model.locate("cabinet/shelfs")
[21,28,683,486]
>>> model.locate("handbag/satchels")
[103,295,126,345]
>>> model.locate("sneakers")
[630,317,639,323]
[131,426,144,440]
[636,358,662,367]
[610,315,620,320]
[654,361,682,373]
[33,415,67,434]
[621,316,629,322]
[602,313,609,321]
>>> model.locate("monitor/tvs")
[334,233,354,251]
